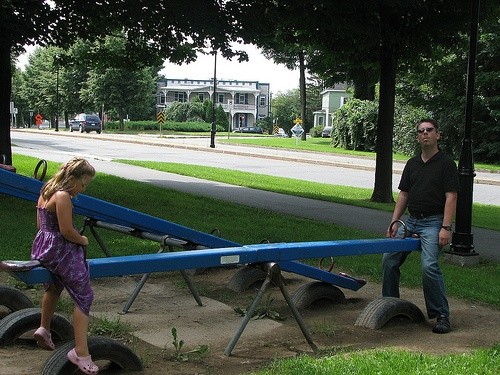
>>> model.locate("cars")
[272,127,285,135]
[235,126,259,133]
[322,126,333,138]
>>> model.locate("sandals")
[66,348,100,374]
[34,326,56,350]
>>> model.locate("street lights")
[210,51,217,148]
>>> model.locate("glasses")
[418,127,435,133]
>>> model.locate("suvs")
[69,113,102,134]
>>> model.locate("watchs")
[441,225,453,231]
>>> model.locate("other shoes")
[435,318,451,333]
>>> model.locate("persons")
[381,116,460,334]
[32,157,99,375]
